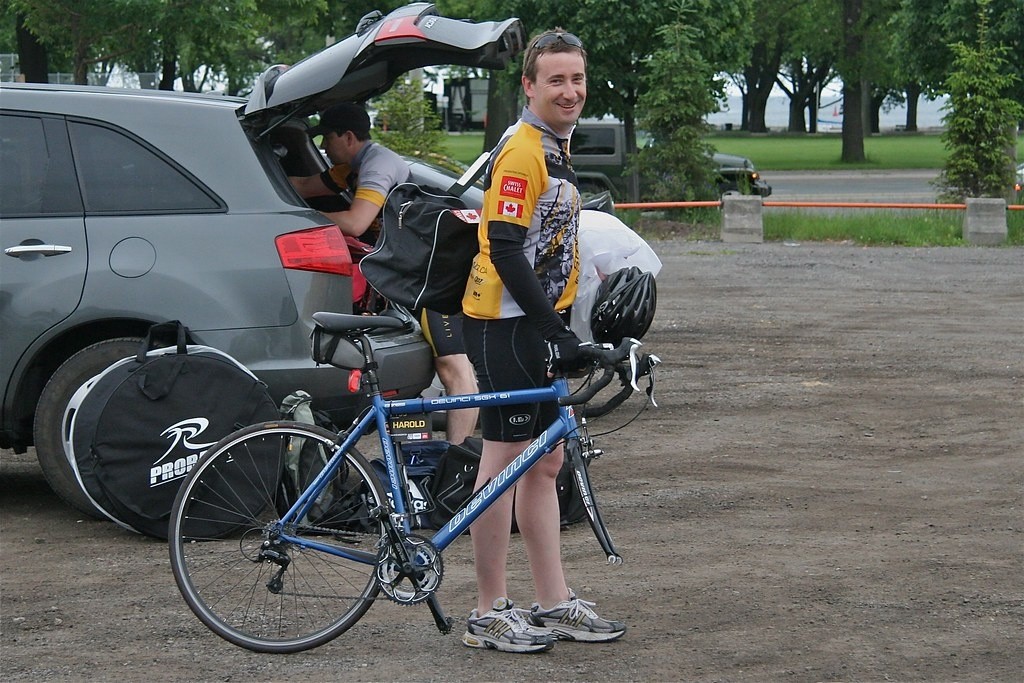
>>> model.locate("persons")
[283,105,482,444]
[460,27,626,654]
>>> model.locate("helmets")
[591,266,658,346]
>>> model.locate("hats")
[305,101,370,137]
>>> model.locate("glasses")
[533,31,583,50]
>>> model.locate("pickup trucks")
[570,120,773,210]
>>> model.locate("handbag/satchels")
[361,430,451,531]
[61,320,287,543]
[434,429,590,535]
[359,153,494,316]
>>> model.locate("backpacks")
[276,387,359,535]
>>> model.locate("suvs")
[0,0,530,523]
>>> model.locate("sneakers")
[530,587,626,642]
[462,597,555,653]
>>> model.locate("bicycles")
[166,314,661,654]
